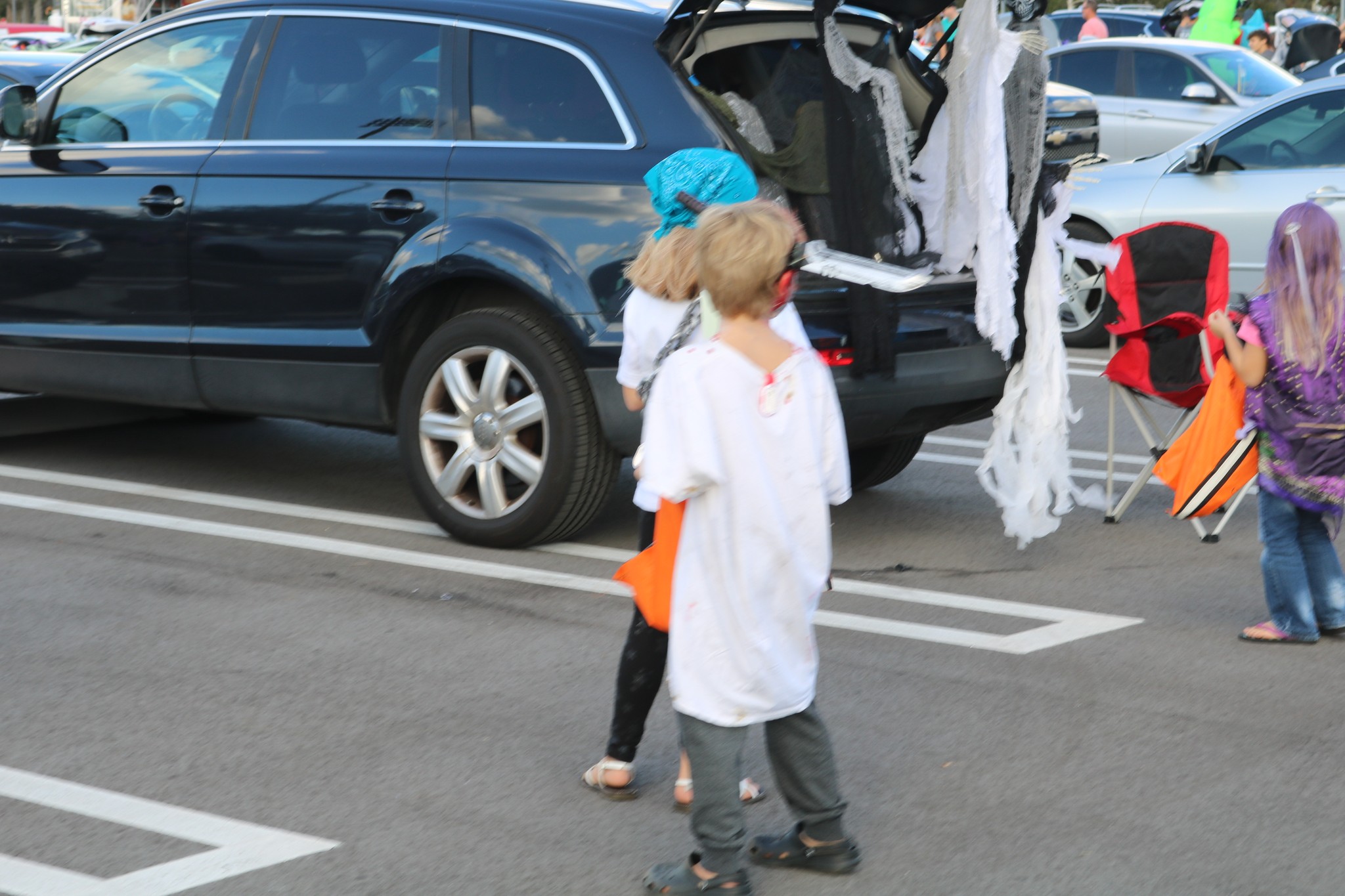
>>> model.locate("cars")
[1036,71,1345,348]
[0,16,224,152]
[903,0,1345,169]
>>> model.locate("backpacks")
[1245,295,1345,514]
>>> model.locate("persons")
[1076,0,1110,43]
[1208,203,1345,643]
[915,4,961,65]
[635,200,862,896]
[585,146,810,814]
[1175,3,1345,73]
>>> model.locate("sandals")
[673,775,767,810]
[748,821,863,874]
[1238,618,1302,642]
[583,756,641,799]
[642,850,752,896]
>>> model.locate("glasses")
[775,246,807,284]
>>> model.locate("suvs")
[0,1,1026,552]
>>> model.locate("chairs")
[1095,220,1234,538]
